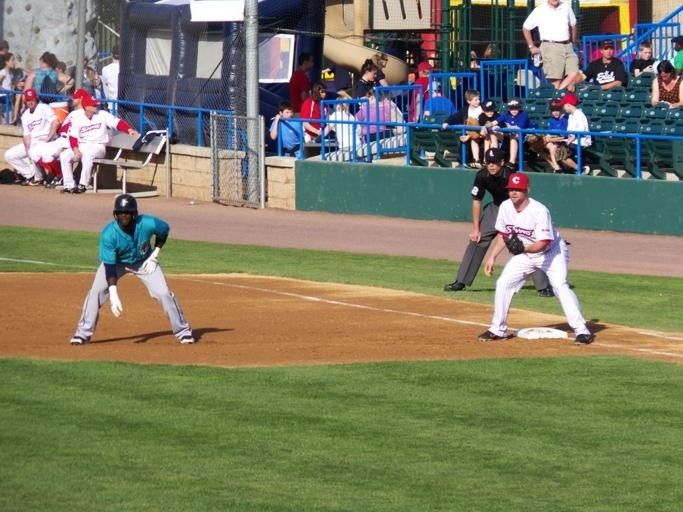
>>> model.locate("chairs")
[410,72,683,181]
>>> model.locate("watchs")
[527,44,534,49]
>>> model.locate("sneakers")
[21,176,86,194]
[539,287,553,297]
[478,331,507,341]
[443,284,466,291]
[574,333,593,344]
[73,337,87,346]
[179,336,193,345]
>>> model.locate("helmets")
[115,195,139,211]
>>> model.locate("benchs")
[82,130,166,198]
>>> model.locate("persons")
[269,53,455,158]
[69,194,196,345]
[475,174,596,343]
[443,148,559,296]
[443,89,592,172]
[471,44,501,68]
[0,39,143,195]
[521,0,581,91]
[584,36,683,109]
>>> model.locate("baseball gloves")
[503,229,524,254]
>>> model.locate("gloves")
[143,247,160,272]
[108,286,123,317]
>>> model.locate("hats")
[485,147,505,163]
[25,88,37,101]
[82,95,101,108]
[506,172,530,192]
[69,88,88,98]
[601,41,615,51]
[507,97,521,110]
[483,99,496,112]
[548,93,579,112]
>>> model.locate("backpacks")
[40,77,57,104]
